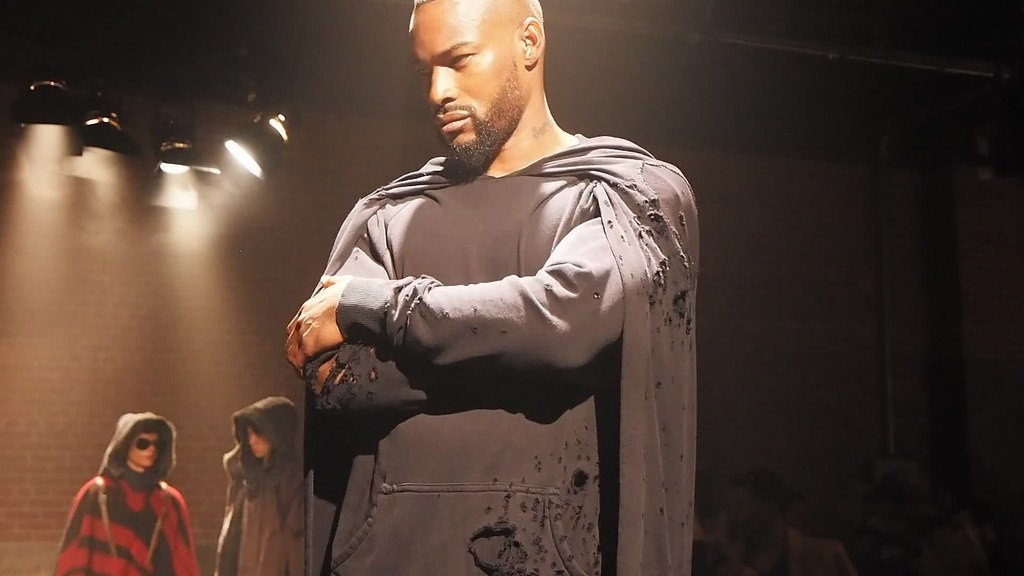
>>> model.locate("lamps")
[10,64,302,181]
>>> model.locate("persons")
[215,397,306,576]
[285,0,700,576]
[51,412,200,575]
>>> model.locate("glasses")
[136,439,162,450]
